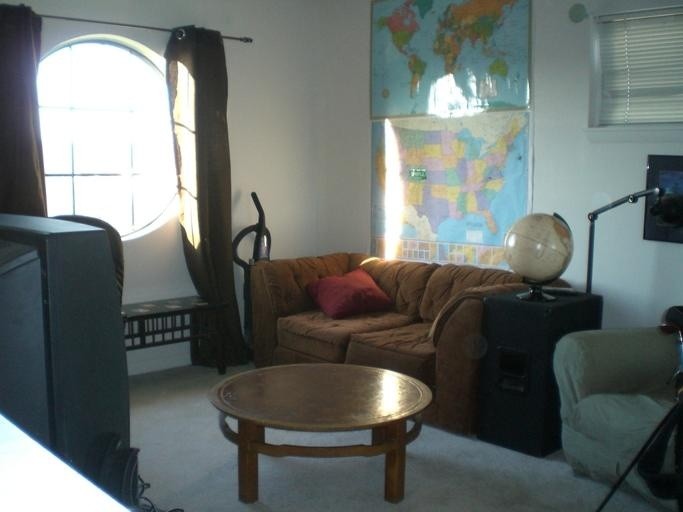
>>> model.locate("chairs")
[552,325,683,511]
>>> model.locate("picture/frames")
[643,153,683,244]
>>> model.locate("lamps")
[587,188,683,293]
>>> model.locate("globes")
[502,210,576,303]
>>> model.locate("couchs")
[250,251,574,438]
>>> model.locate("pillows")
[306,267,393,320]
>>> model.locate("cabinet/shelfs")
[474,292,603,458]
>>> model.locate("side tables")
[121,295,229,374]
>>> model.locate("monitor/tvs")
[0,214,130,507]
